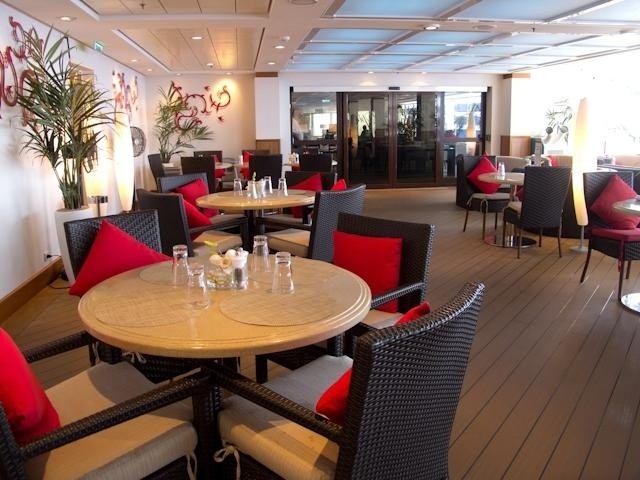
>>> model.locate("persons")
[360,125,369,136]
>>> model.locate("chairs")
[455,155,511,240]
[502,166,571,259]
[579,171,639,303]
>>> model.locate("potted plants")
[15,28,91,287]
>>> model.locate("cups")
[169,243,191,275]
[232,175,289,200]
[497,162,507,177]
[184,261,211,310]
[268,251,294,297]
[250,235,270,275]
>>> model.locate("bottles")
[210,248,250,292]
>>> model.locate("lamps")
[568,96,592,254]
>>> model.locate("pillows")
[467,157,503,194]
[591,176,640,229]
